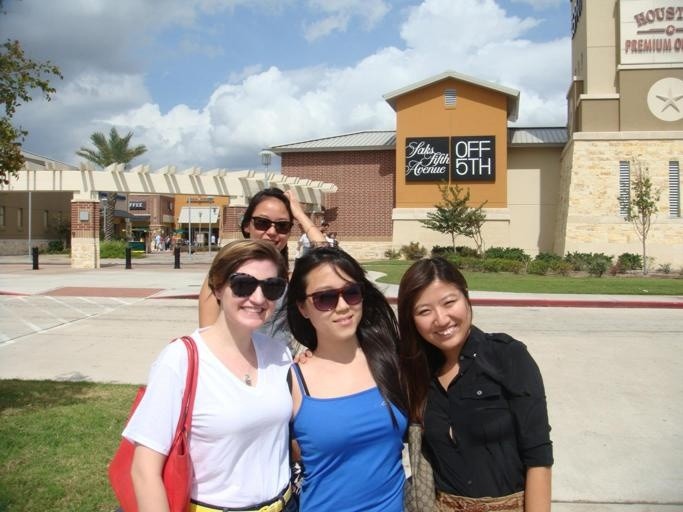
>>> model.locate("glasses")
[248,216,295,237]
[303,282,364,312]
[228,271,287,302]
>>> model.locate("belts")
[190,480,292,512]
[435,494,519,512]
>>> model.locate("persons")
[293,256,554,512]
[297,223,339,255]
[154,234,171,252]
[121,239,298,512]
[288,246,410,512]
[198,187,328,328]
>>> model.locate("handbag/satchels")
[403,424,436,512]
[106,334,201,512]
